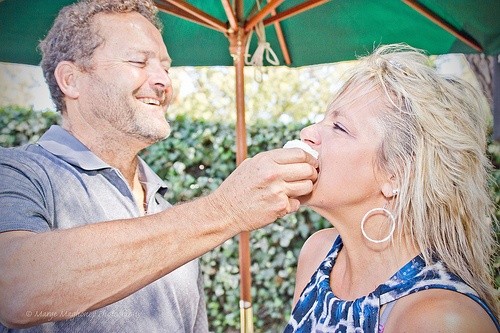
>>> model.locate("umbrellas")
[0,0,500,333]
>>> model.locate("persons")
[0,0,318,333]
[149,44,500,333]
